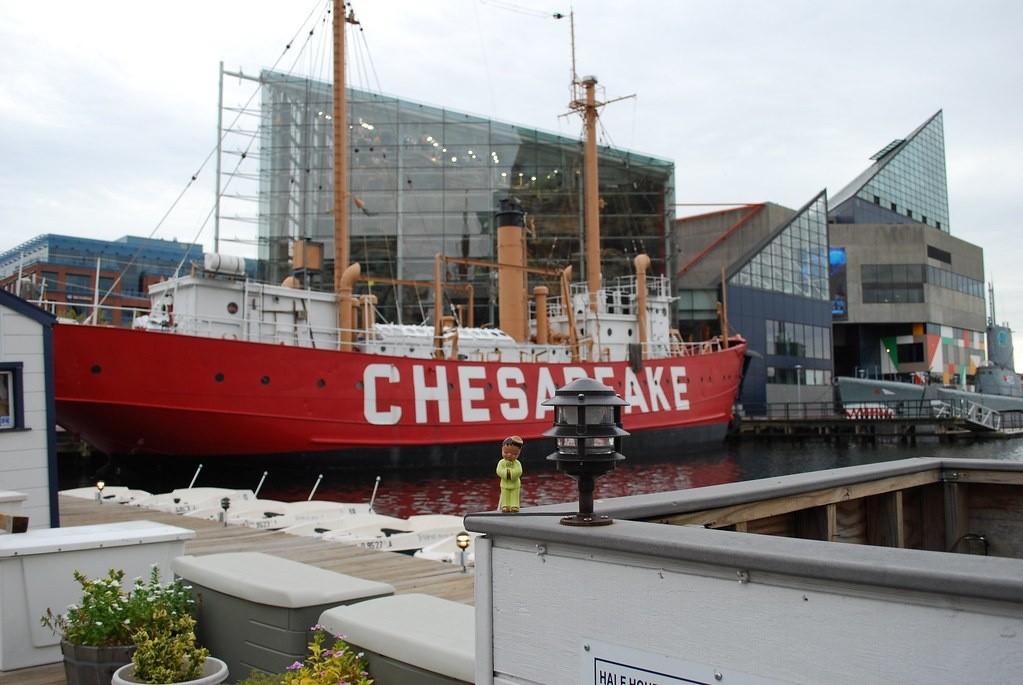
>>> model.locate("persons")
[497,436,522,512]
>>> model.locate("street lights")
[794,364,803,418]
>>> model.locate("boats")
[829,282,1023,437]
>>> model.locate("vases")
[59,639,136,685]
[111,655,229,685]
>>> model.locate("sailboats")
[48,0,750,474]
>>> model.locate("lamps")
[540,376,631,526]
[221,497,231,527]
[455,532,470,574]
[96,481,105,504]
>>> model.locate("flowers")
[37,563,374,685]
